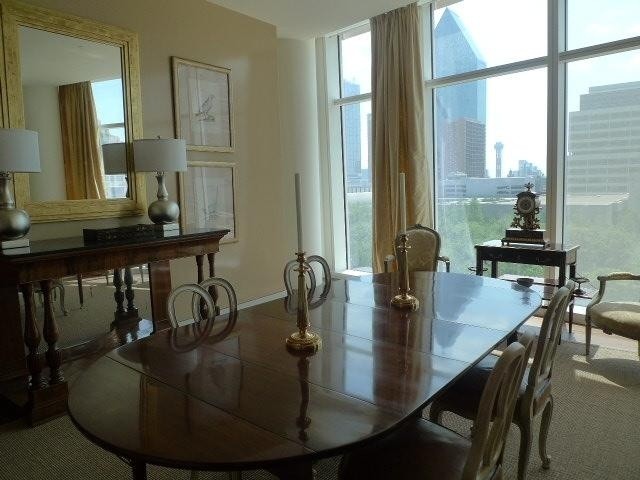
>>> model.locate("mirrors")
[1,0,151,224]
[17,262,156,374]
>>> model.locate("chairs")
[165,276,237,327]
[284,281,332,314]
[283,255,332,294]
[169,311,238,354]
[383,224,452,273]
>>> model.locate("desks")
[2,225,228,426]
[63,270,543,480]
[474,240,580,345]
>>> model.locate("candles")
[293,173,305,256]
[399,171,407,234]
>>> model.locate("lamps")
[102,142,129,198]
[0,128,42,241]
[134,135,188,225]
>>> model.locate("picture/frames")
[168,55,240,244]
